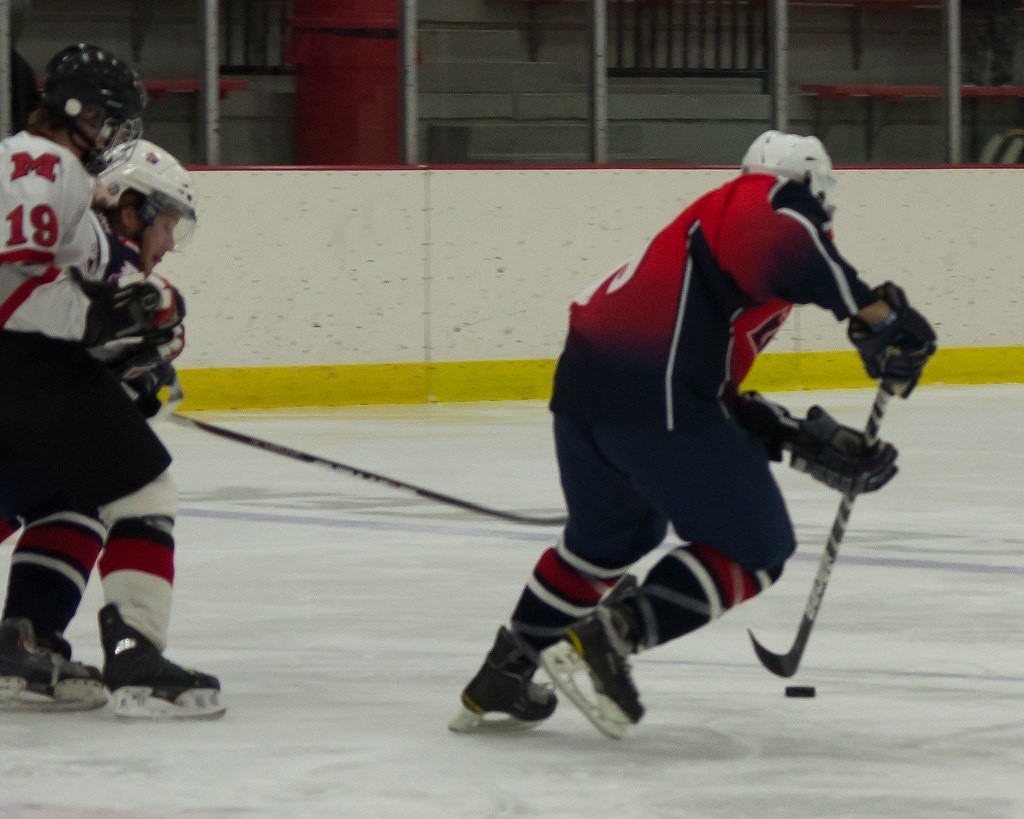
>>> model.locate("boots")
[536,574,648,738]
[448,625,557,732]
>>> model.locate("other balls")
[785,686,815,699]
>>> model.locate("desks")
[799,82,1024,166]
[140,76,249,159]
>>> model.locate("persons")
[458,131,940,742]
[1,136,200,545]
[1,42,227,719]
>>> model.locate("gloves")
[846,279,936,399]
[790,405,898,495]
[104,321,186,381]
[109,272,187,342]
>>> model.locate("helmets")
[40,46,149,131]
[92,140,199,223]
[742,129,836,195]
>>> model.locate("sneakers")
[96,602,224,719]
[0,617,108,712]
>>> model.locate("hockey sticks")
[745,347,900,676]
[163,410,570,529]
